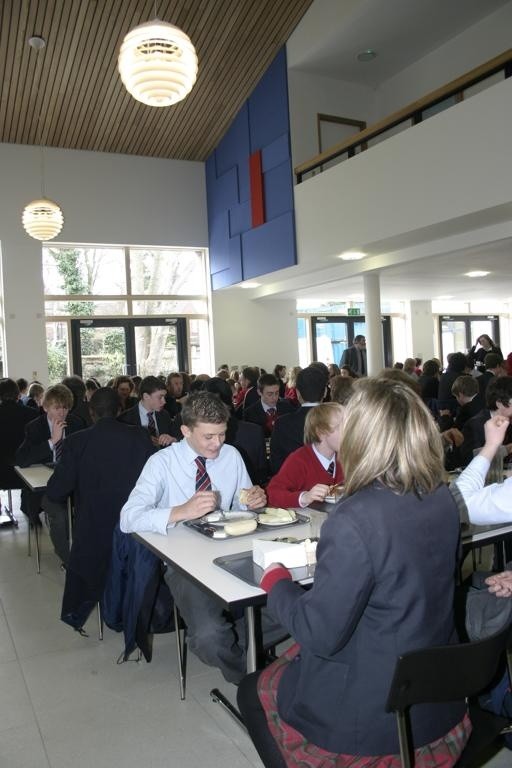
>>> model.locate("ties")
[327,462,334,476]
[195,456,212,492]
[146,412,157,438]
[360,349,365,375]
[267,408,275,416]
[54,438,64,462]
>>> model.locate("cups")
[472,447,506,487]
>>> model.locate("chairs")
[54,549,106,643]
[20,505,47,573]
[118,573,187,699]
[386,614,512,766]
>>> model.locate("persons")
[1,331,511,711]
[238,380,473,768]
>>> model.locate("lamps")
[19,195,67,244]
[113,1,201,113]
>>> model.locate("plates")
[199,506,259,526]
[255,515,299,526]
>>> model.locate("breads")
[239,489,253,505]
[259,507,296,523]
[224,520,257,535]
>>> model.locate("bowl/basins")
[324,484,345,504]
